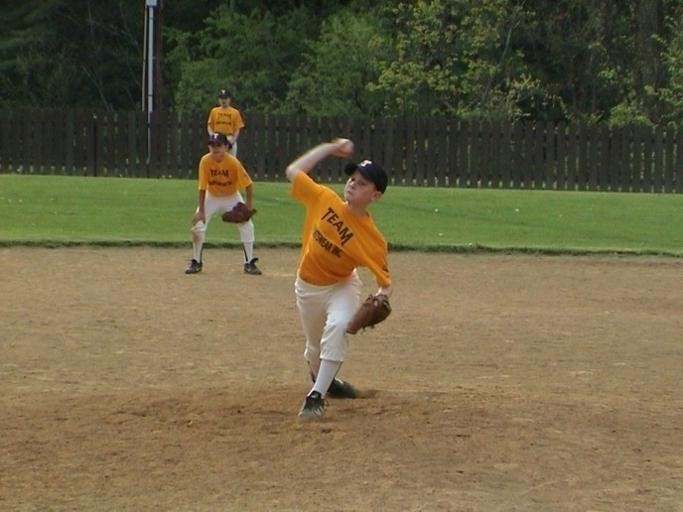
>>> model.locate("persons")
[206,89,245,158]
[183,132,262,275]
[284,138,394,420]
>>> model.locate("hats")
[208,131,227,145]
[216,90,230,98]
[345,160,389,194]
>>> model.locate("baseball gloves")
[346,294,391,335]
[221,202,251,222]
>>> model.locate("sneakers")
[299,391,327,416]
[328,377,358,399]
[244,257,262,275]
[184,259,202,273]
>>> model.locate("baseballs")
[338,138,354,155]
